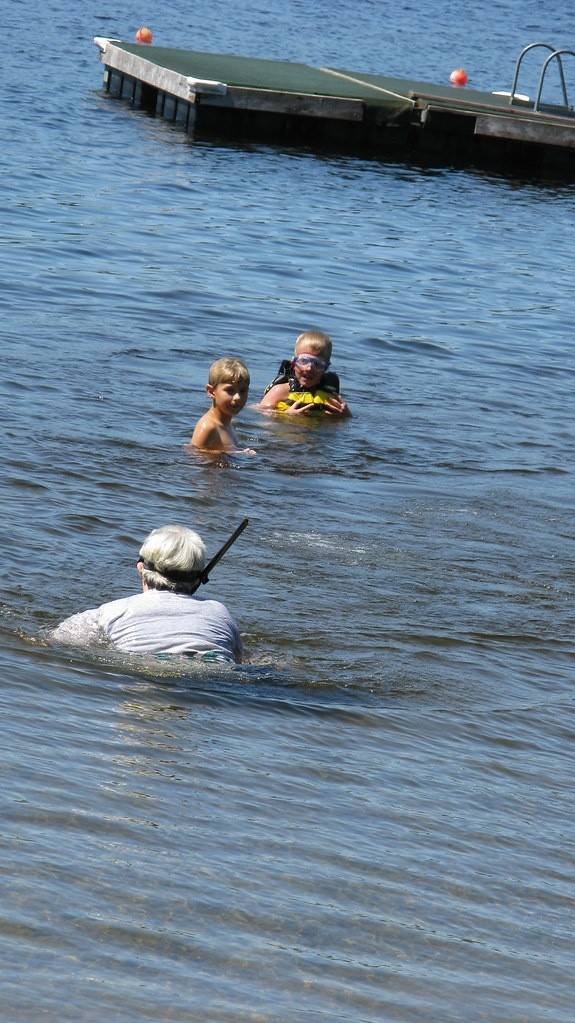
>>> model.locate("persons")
[190,357,250,449]
[259,330,354,418]
[50,524,243,667]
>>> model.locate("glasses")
[293,353,329,372]
